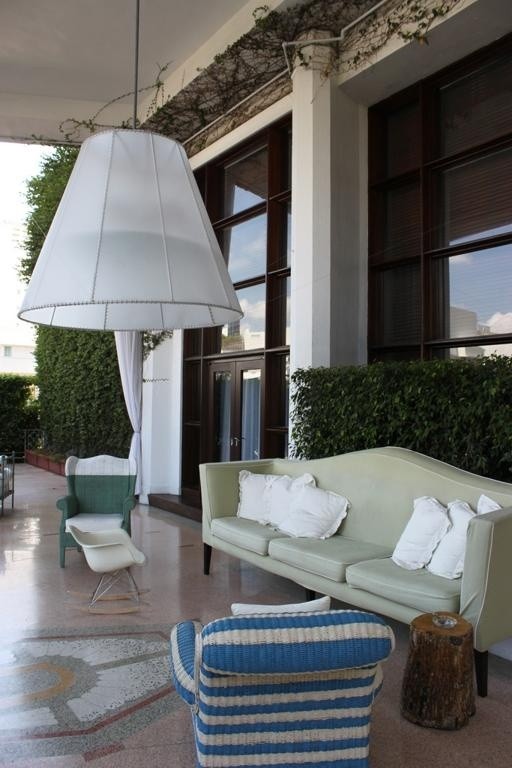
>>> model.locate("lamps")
[14,1,246,336]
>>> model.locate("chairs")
[166,608,396,768]
[56,450,152,615]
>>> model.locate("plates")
[433,615,457,629]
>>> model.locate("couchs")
[196,445,511,699]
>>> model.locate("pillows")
[235,467,350,542]
[390,494,501,582]
[230,596,332,616]
